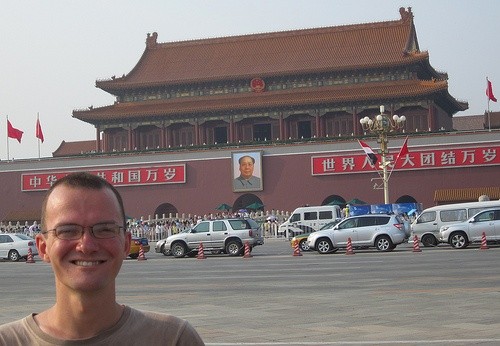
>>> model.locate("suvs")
[155,217,264,257]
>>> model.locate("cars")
[0,233,38,262]
[291,214,411,254]
[128,234,150,258]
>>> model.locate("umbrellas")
[246,202,265,211]
[408,208,417,215]
[346,198,366,204]
[216,203,233,210]
[328,200,343,204]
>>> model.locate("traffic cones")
[137,244,147,260]
[197,243,207,259]
[25,247,35,263]
[346,237,355,254]
[412,235,422,252]
[293,240,303,256]
[243,241,253,258]
[481,232,489,249]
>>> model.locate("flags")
[359,140,378,165]
[394,138,408,159]
[485,81,497,102]
[35,119,44,143]
[7,120,24,143]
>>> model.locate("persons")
[0,225,40,236]
[401,212,416,224]
[127,211,278,241]
[0,173,204,346]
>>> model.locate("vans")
[411,200,500,248]
[278,205,341,240]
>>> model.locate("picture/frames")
[230,149,264,192]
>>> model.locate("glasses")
[41,222,126,240]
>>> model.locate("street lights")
[360,105,406,204]
